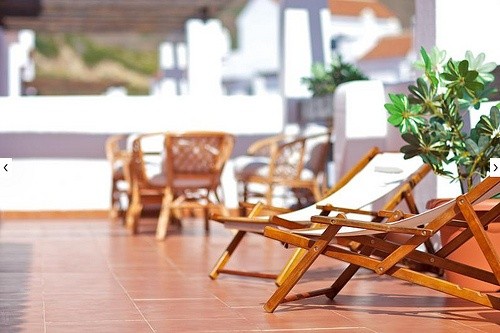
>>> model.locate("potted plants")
[384,44,500,292]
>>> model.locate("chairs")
[105,123,500,313]
